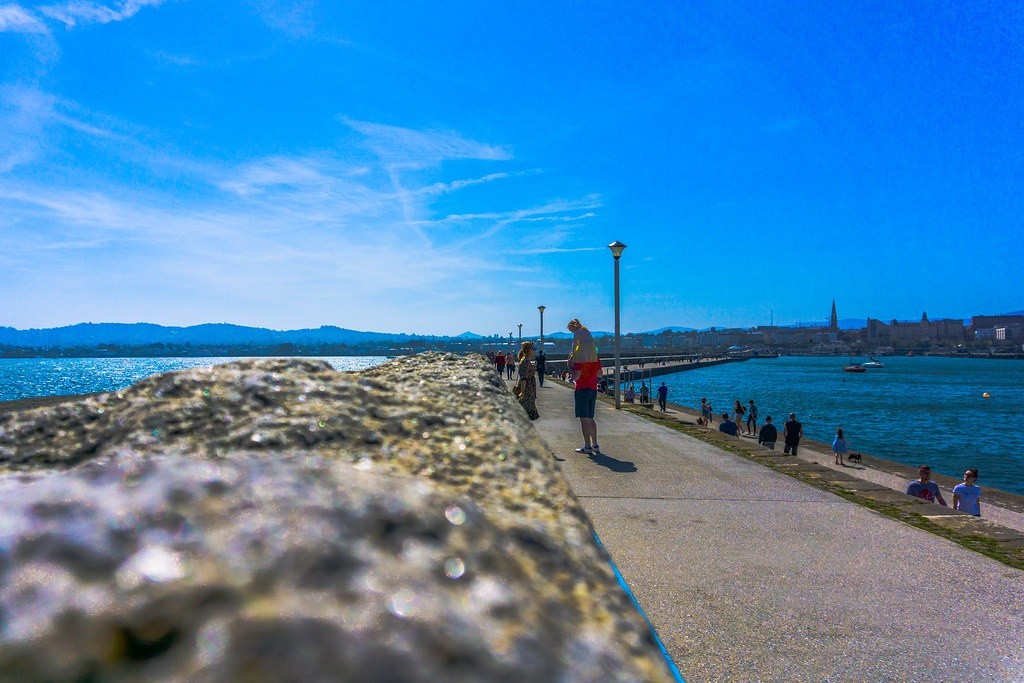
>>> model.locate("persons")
[758,415,778,450]
[730,400,745,435]
[534,349,547,388]
[486,350,515,380]
[597,376,608,393]
[656,382,668,412]
[718,412,739,438]
[701,398,710,428]
[567,318,603,454]
[782,412,803,457]
[627,386,636,404]
[552,367,575,385]
[831,429,847,465]
[639,359,646,368]
[746,399,758,436]
[640,383,649,403]
[512,341,540,422]
[905,466,947,507]
[951,468,981,517]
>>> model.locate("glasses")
[922,471,931,475]
[964,472,974,478]
[529,346,535,350]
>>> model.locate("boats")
[842,360,866,372]
[863,355,884,368]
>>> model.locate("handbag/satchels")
[512,384,519,395]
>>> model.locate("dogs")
[848,453,862,463]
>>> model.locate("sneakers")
[575,446,593,454]
[592,445,599,453]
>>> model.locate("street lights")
[537,305,546,348]
[509,332,512,349]
[608,241,627,410]
[517,324,523,349]
